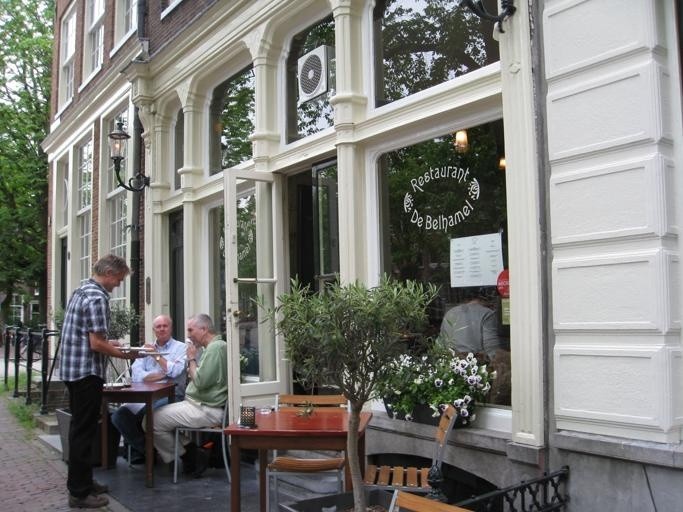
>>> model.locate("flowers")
[375,344,500,422]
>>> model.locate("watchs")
[154,354,163,361]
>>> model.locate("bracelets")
[186,358,196,367]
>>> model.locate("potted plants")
[250,269,444,512]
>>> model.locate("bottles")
[106,366,114,391]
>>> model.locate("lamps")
[107,121,151,192]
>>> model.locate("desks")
[101,380,180,489]
[223,408,373,512]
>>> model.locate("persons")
[433,285,502,369]
[55,253,152,510]
[139,311,228,480]
[107,313,192,468]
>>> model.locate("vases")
[382,396,475,429]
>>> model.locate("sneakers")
[184,444,211,478]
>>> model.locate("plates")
[114,347,154,351]
[103,383,125,388]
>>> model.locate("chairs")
[173,400,233,484]
[266,392,458,502]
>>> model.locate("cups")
[122,377,133,388]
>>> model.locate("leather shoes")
[91,480,108,494]
[68,493,109,508]
